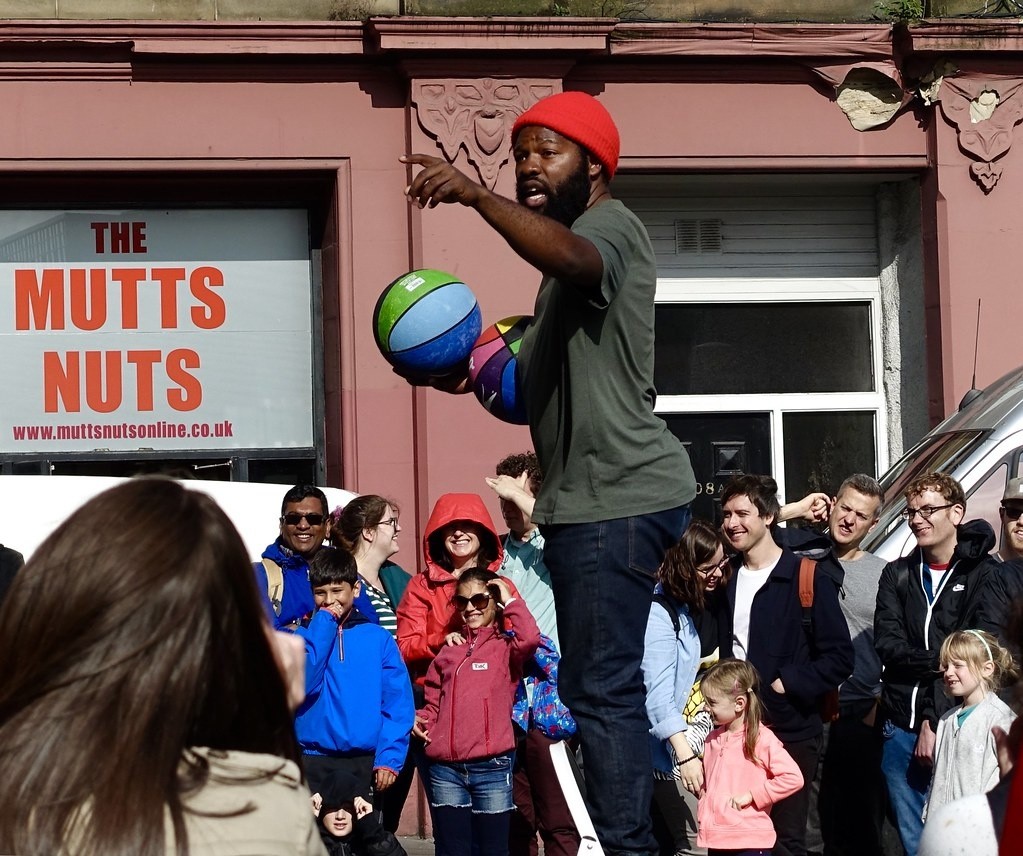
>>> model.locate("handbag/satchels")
[799,555,846,722]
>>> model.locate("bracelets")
[677,754,698,765]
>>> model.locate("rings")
[687,782,693,785]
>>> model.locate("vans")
[821,365,1023,563]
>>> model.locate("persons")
[397,93,700,855]
[484,451,605,856]
[293,547,415,813]
[718,473,1023,856]
[409,568,541,856]
[395,492,526,707]
[638,518,724,855]
[1,476,331,856]
[696,657,804,856]
[251,484,380,632]
[308,773,408,856]
[333,494,418,855]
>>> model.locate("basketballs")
[468,315,534,425]
[371,267,484,376]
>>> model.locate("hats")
[511,91,620,184]
[1003,477,1023,500]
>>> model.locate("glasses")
[279,514,326,525]
[903,504,955,520]
[452,593,493,611]
[363,517,397,528]
[1005,507,1023,519]
[695,554,730,580]
